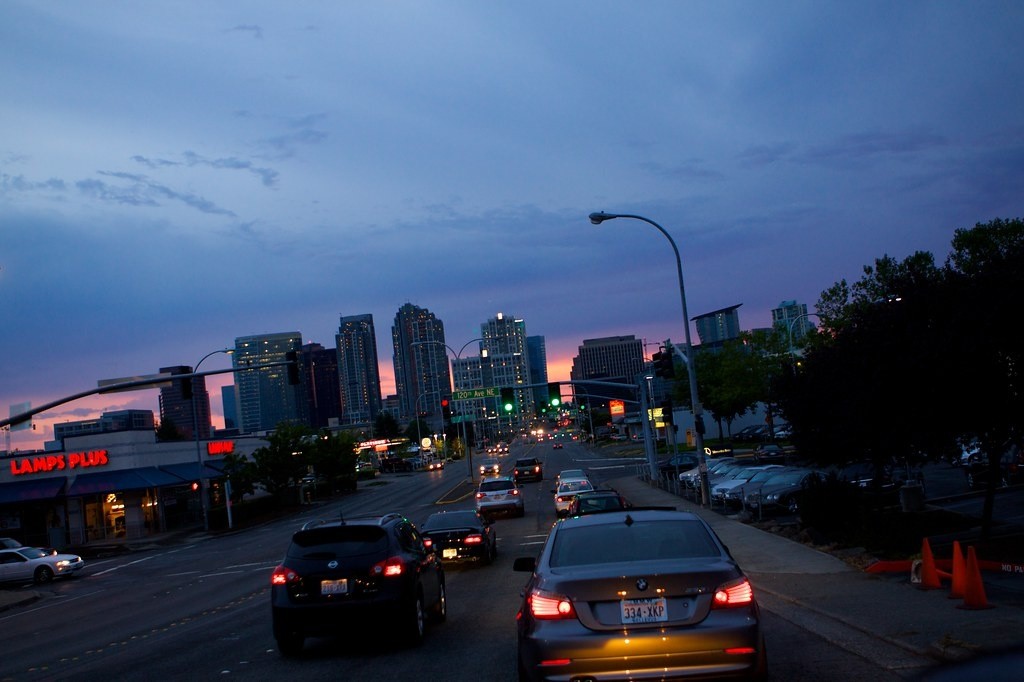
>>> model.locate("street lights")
[916,539,949,591]
[414,391,440,470]
[190,348,237,531]
[645,376,660,485]
[411,335,503,477]
[588,212,712,508]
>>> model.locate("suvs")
[472,476,526,525]
[269,510,448,659]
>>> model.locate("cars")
[520,428,581,450]
[497,441,510,454]
[551,468,634,519]
[610,433,645,441]
[513,456,543,485]
[428,459,445,471]
[419,508,497,572]
[511,505,770,682]
[655,425,1024,532]
[479,456,502,475]
[0,537,85,587]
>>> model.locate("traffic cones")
[946,540,969,600]
[954,546,997,611]
[910,559,953,587]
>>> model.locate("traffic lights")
[547,382,562,408]
[485,396,497,419]
[441,399,451,419]
[501,387,516,414]
